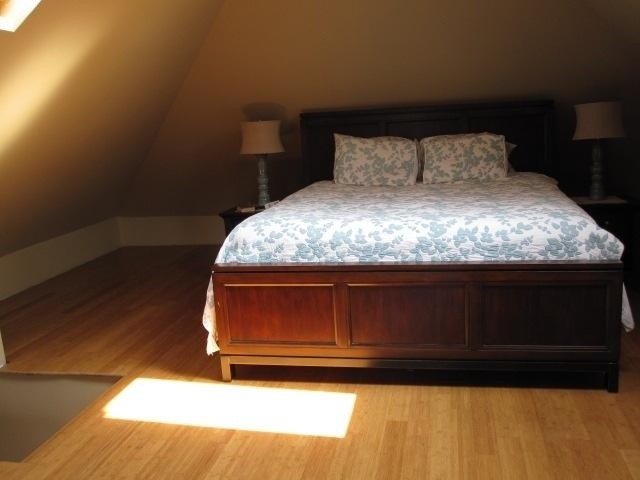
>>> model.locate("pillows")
[331,130,517,189]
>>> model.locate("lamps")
[571,100,629,202]
[239,119,288,210]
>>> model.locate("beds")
[210,99,626,394]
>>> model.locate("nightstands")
[567,196,639,289]
[217,205,265,237]
